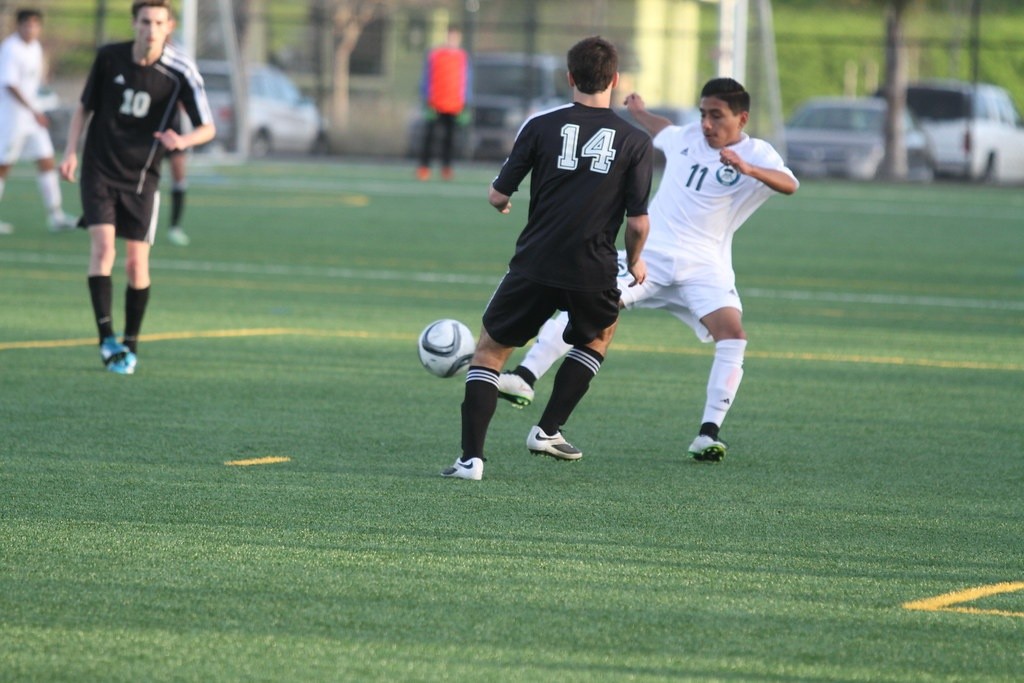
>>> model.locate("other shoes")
[48,217,80,232]
[170,229,189,245]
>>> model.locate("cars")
[37,60,96,149]
[780,95,937,186]
[189,58,332,161]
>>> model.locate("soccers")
[418,318,476,378]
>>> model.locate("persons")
[415,25,476,183]
[0,8,77,232]
[148,12,193,246]
[438,38,653,483]
[496,76,801,464]
[60,0,216,375]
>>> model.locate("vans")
[409,49,574,162]
[874,78,1024,185]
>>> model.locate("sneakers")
[497,372,534,408]
[440,456,483,482]
[101,337,137,375]
[527,425,582,463]
[688,434,725,462]
